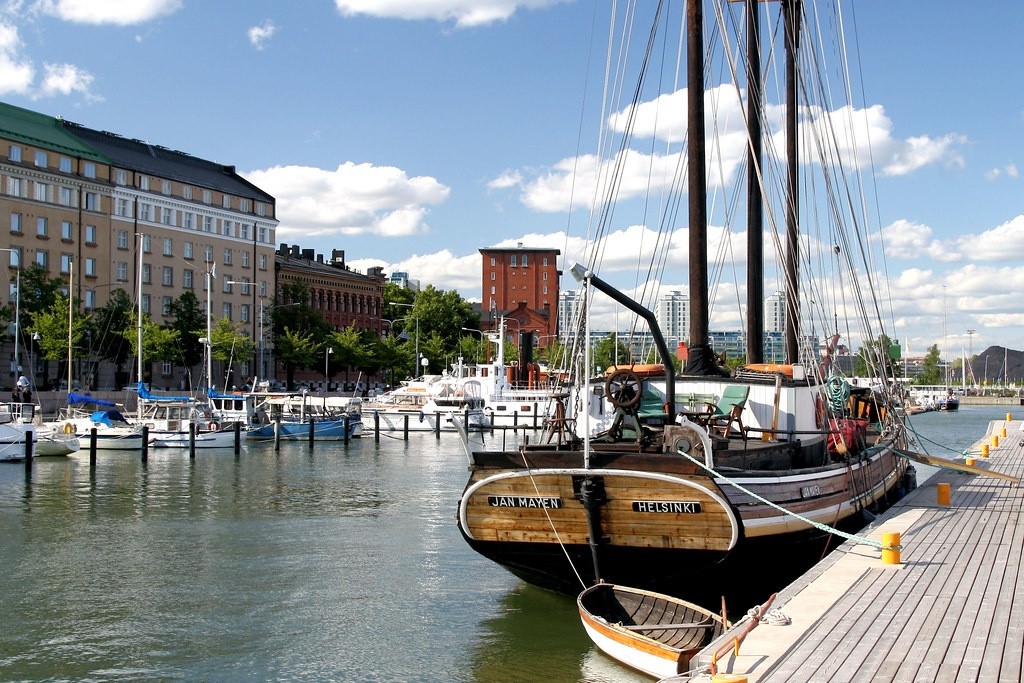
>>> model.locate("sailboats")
[452,0,922,598]
[0,231,591,463]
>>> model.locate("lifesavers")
[208,421,220,431]
[744,363,793,379]
[64,423,73,434]
[606,363,666,376]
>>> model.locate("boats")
[852,378,961,414]
[574,578,740,681]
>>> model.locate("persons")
[22,386,32,402]
[17,374,30,392]
[12,389,21,403]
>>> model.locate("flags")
[906,343,910,352]
